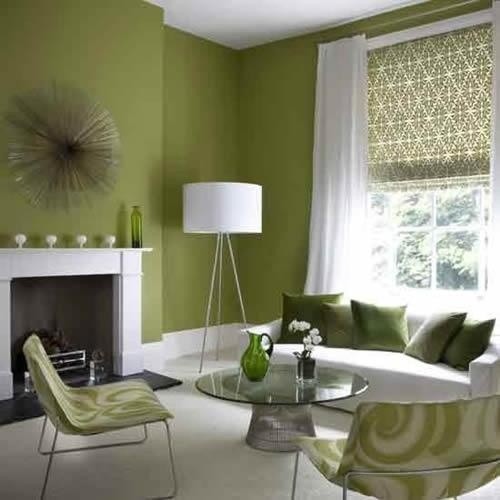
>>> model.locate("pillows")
[404,310,468,364]
[440,317,496,370]
[349,299,410,353]
[275,291,344,344]
[317,303,352,349]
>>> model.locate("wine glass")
[45,235,56,249]
[15,234,26,248]
[105,236,116,248]
[76,235,86,248]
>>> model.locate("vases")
[292,350,316,381]
[296,384,317,403]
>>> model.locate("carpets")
[0,368,184,425]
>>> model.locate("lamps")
[180,181,264,374]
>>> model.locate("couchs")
[241,307,499,415]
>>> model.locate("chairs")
[288,393,499,500]
[19,333,179,500]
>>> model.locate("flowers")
[287,320,322,353]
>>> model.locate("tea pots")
[240,330,275,382]
